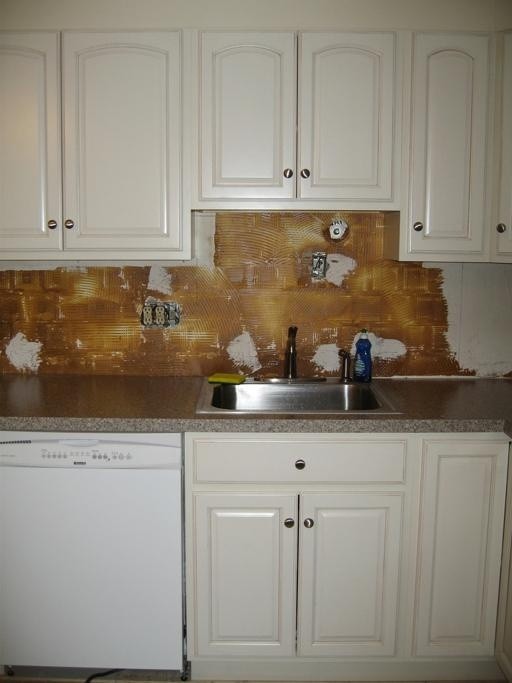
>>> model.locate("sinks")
[196,378,404,414]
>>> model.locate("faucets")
[338,348,354,383]
[284,324,299,379]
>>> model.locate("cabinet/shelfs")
[412,433,509,668]
[379,25,490,261]
[184,431,413,665]
[490,24,511,264]
[0,26,195,262]
[194,27,407,215]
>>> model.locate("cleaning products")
[353,327,373,382]
